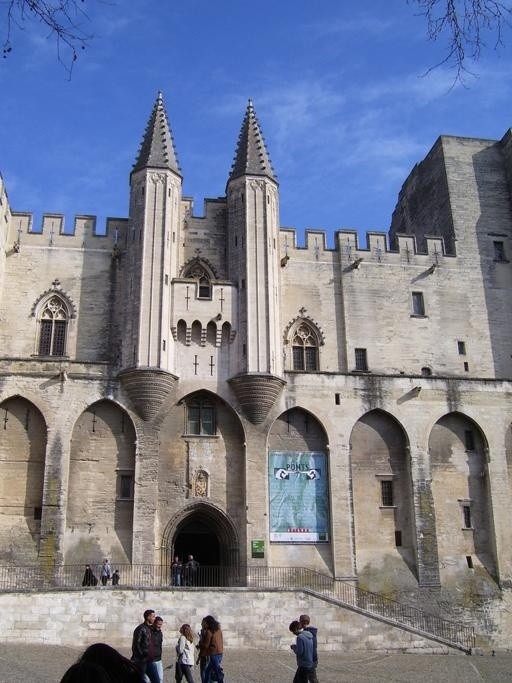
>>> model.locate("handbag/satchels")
[175,662,182,680]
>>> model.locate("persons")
[288,620,313,683]
[83,565,93,586]
[299,615,319,683]
[99,559,110,586]
[170,554,200,587]
[110,569,120,585]
[58,609,224,683]
